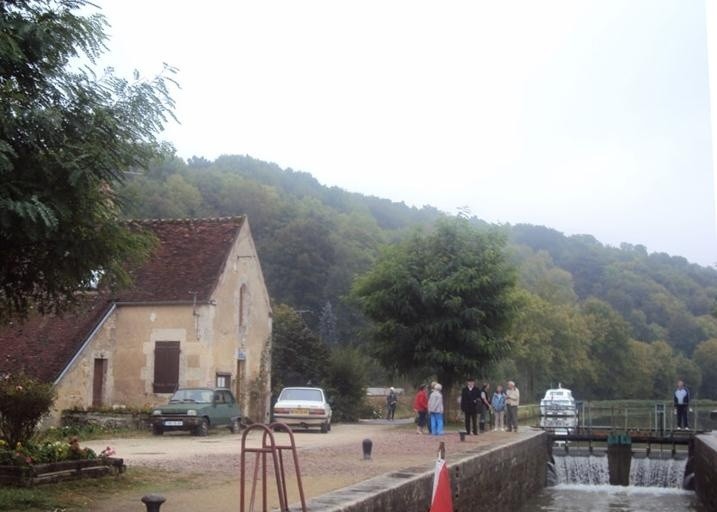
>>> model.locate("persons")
[673,380,690,432]
[428,383,445,435]
[413,383,428,435]
[386,386,396,421]
[479,384,492,432]
[425,381,436,434]
[462,378,481,434]
[491,384,506,432]
[504,381,520,432]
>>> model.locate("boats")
[538,415,578,446]
[539,381,578,417]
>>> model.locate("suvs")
[149,386,240,436]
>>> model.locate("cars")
[271,385,333,432]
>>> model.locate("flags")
[430,450,454,512]
